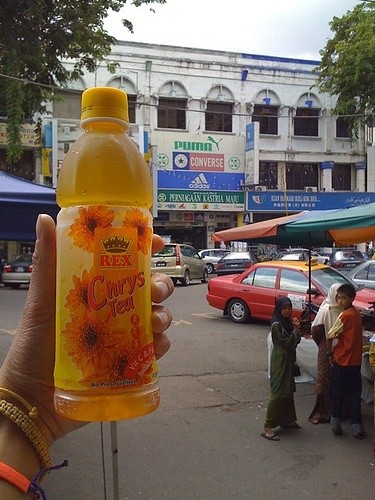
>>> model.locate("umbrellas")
[212,201,375,336]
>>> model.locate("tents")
[0,171,61,242]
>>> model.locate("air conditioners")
[304,187,317,192]
[254,186,266,191]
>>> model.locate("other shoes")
[280,422,302,429]
[261,432,280,441]
[330,418,344,434]
[352,429,367,439]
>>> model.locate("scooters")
[299,301,375,334]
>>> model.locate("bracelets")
[0,460,68,500]
[0,386,51,479]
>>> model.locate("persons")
[262,296,300,440]
[327,283,366,439]
[310,282,344,424]
[0,214,175,500]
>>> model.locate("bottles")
[52,86,160,422]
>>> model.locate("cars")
[191,248,232,274]
[150,243,209,286]
[2,253,33,290]
[216,251,261,276]
[270,247,375,291]
[206,258,375,331]
